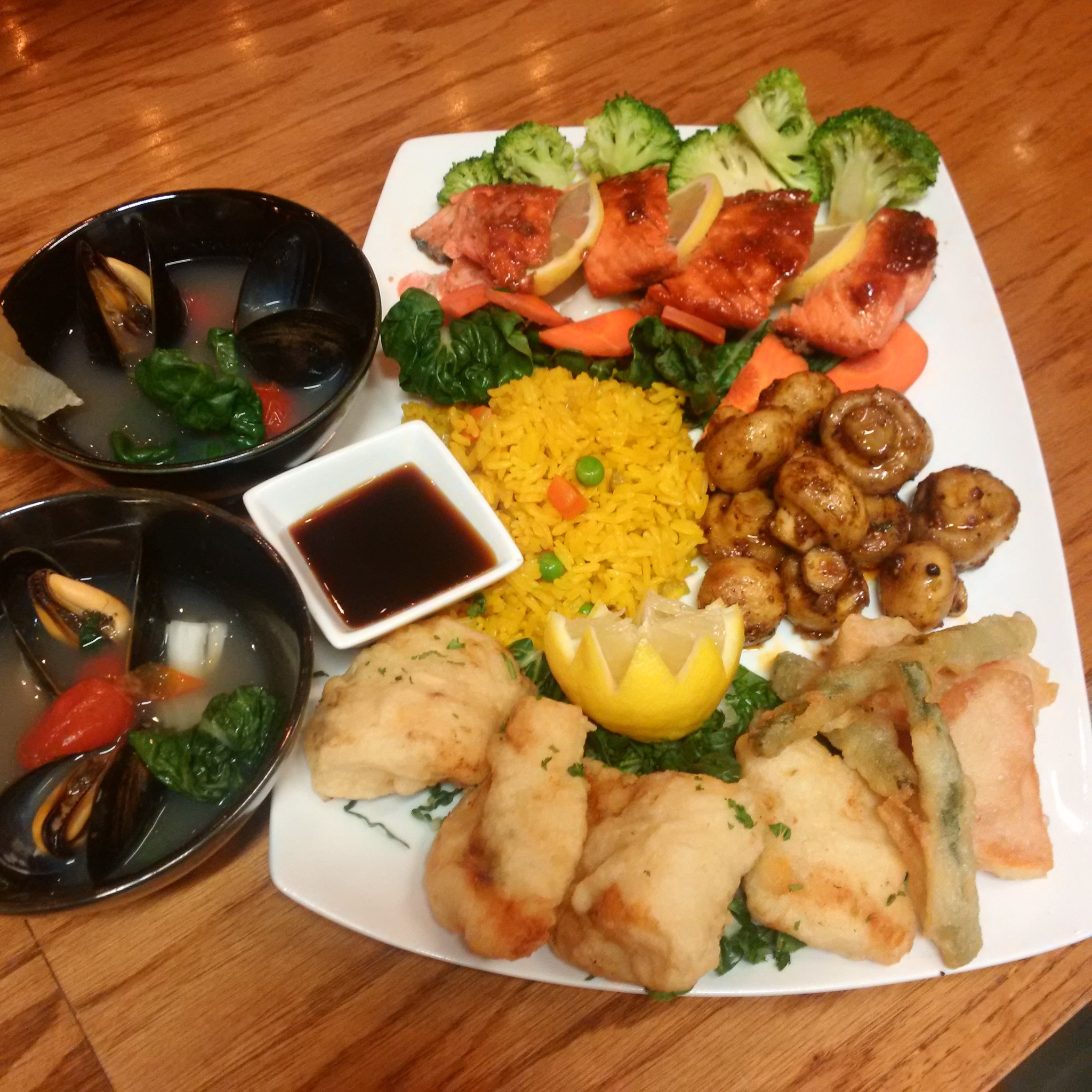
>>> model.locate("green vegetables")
[411,635,810,973]
[109,327,265,470]
[134,682,282,808]
[378,286,846,427]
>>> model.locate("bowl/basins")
[242,418,523,651]
[0,487,314,918]
[0,188,383,500]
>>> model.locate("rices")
[391,363,711,657]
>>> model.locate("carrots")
[429,279,930,431]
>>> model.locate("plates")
[266,125,1091,996]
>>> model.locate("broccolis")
[439,65,943,223]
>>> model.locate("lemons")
[525,179,608,296]
[662,172,726,260]
[539,595,746,740]
[778,216,869,298]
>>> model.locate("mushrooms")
[688,371,1021,649]
